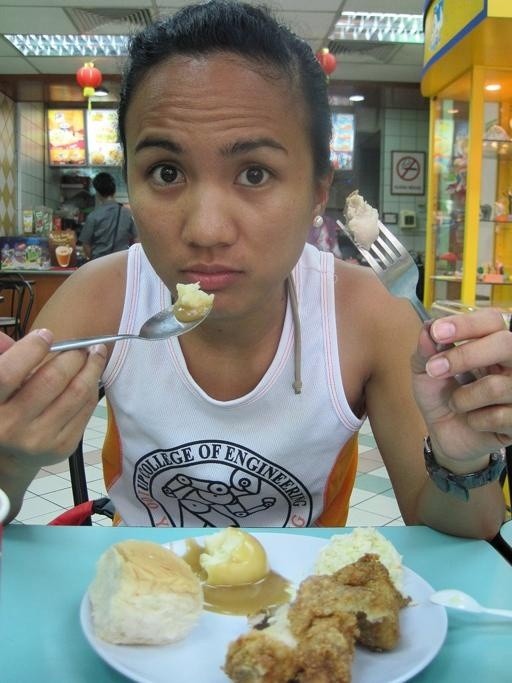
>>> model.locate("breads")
[89,540,205,645]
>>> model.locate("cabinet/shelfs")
[421,20,510,314]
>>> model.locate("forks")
[331,201,480,385]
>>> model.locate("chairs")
[0,270,35,342]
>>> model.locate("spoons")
[37,294,215,352]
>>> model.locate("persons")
[79,171,137,260]
[0,2,510,540]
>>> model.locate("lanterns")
[314,47,337,72]
[75,60,106,87]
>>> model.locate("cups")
[55,245,72,267]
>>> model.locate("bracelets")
[423,435,508,502]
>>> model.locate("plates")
[76,524,452,683]
